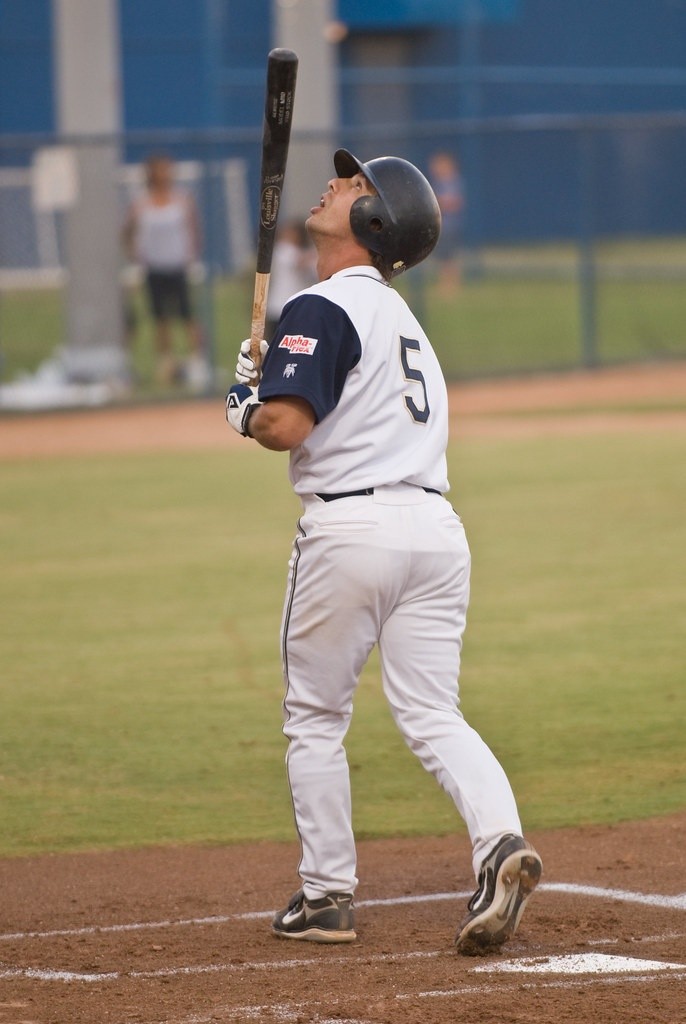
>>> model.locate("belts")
[314,487,442,502]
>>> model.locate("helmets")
[333,148,441,281]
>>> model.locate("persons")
[225,148,542,956]
[120,153,214,391]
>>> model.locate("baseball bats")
[250,47,301,388]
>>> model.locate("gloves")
[234,338,269,388]
[224,382,263,438]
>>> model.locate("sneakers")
[271,886,357,945]
[453,833,543,958]
